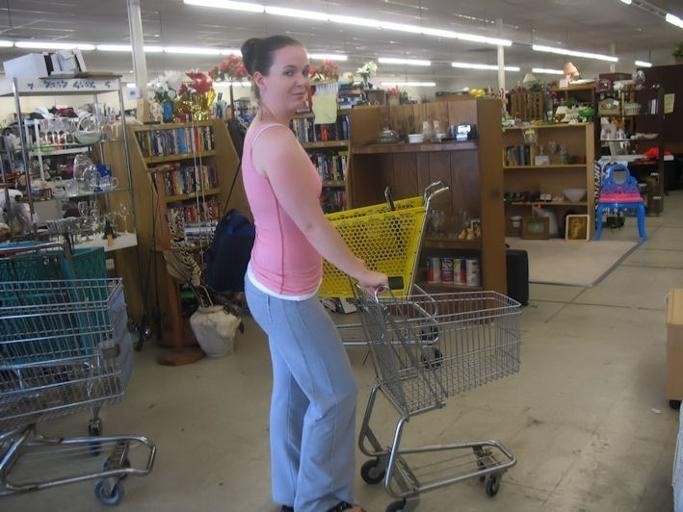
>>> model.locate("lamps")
[621,1,634,6]
[534,44,620,63]
[635,60,654,67]
[666,12,683,29]
[182,0,513,48]
[1,35,350,62]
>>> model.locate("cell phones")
[388,276,404,290]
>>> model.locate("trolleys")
[316,179,449,370]
[0,277,158,507]
[343,267,521,512]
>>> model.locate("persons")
[238,34,391,511]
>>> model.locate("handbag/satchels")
[203,209,255,293]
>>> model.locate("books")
[135,114,351,224]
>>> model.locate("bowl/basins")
[76,131,102,144]
[563,189,586,201]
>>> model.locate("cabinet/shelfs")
[1,88,682,365]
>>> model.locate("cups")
[99,176,119,191]
[408,134,425,144]
[66,180,79,195]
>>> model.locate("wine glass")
[77,201,99,232]
[93,103,118,142]
[120,201,130,237]
[41,119,76,147]
